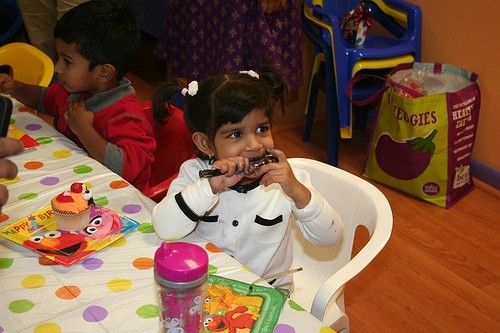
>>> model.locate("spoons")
[199,155,280,180]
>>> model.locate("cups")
[153,241,209,333]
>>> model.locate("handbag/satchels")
[347,60,480,207]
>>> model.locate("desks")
[0,92,337,333]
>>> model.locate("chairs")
[139,100,198,204]
[299,0,422,167]
[285,157,393,333]
[0,42,54,117]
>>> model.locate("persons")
[152,62,344,297]
[157,0,303,109]
[16,0,89,84]
[0,0,157,192]
[0,137,23,210]
